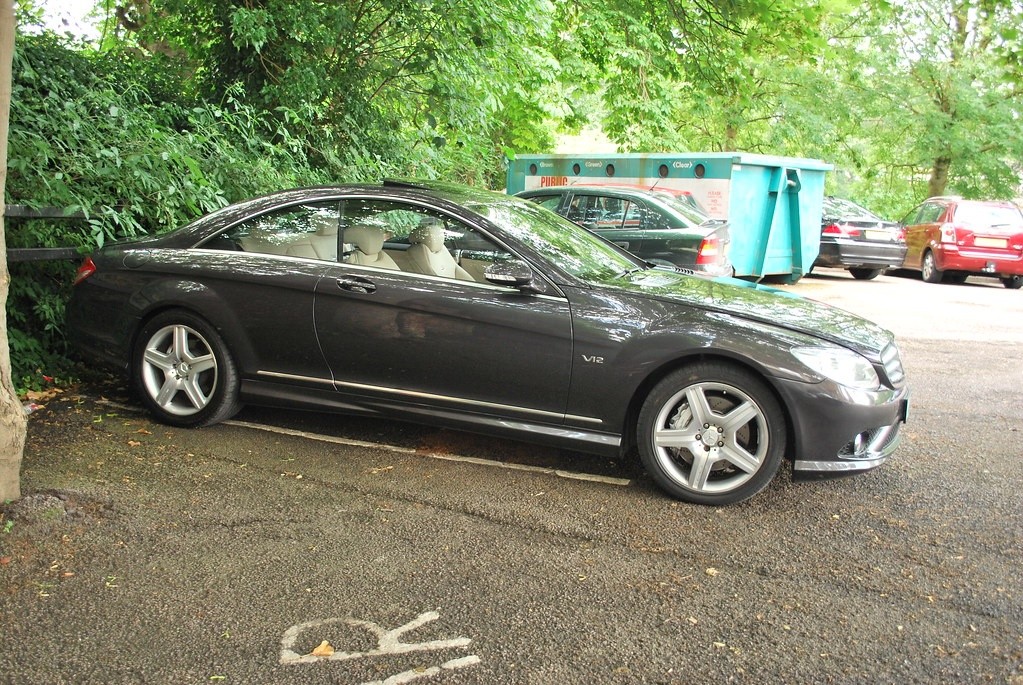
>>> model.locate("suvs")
[881,196,1023,289]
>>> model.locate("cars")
[809,195,909,278]
[64,178,910,507]
[511,186,734,280]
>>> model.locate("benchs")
[237,211,355,261]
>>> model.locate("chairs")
[343,226,401,270]
[404,225,476,282]
